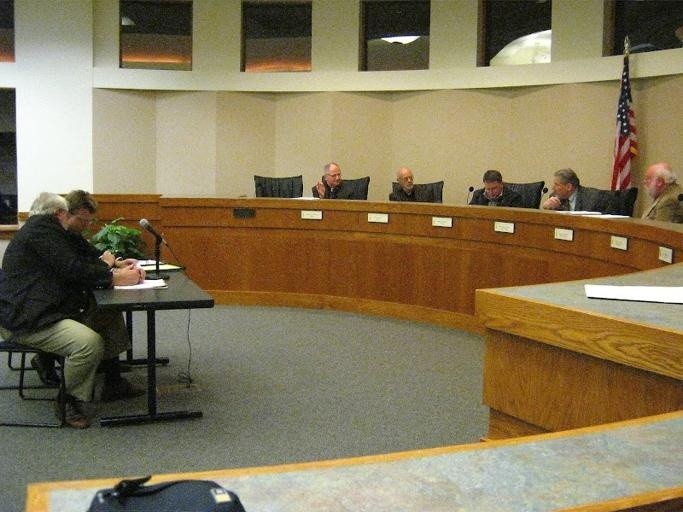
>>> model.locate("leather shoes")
[102,377,147,402]
[54,397,90,430]
[30,351,60,388]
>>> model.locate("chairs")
[0,267,95,428]
[678,193,683,223]
[254,174,303,198]
[0,351,64,390]
[503,181,545,209]
[322,175,370,200]
[392,181,444,203]
[600,187,638,217]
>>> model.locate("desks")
[89,257,214,427]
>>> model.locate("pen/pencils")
[97,250,111,260]
[140,263,166,267]
[552,194,567,209]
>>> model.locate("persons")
[0,190,145,430]
[28,188,146,405]
[467,169,523,208]
[387,166,433,204]
[311,162,355,200]
[638,162,683,224]
[541,167,604,213]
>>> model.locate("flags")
[609,54,638,192]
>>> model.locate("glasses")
[69,208,97,228]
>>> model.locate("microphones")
[467,187,474,204]
[139,218,168,245]
[535,188,548,209]
[672,194,683,223]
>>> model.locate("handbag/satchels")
[86,473,245,512]
